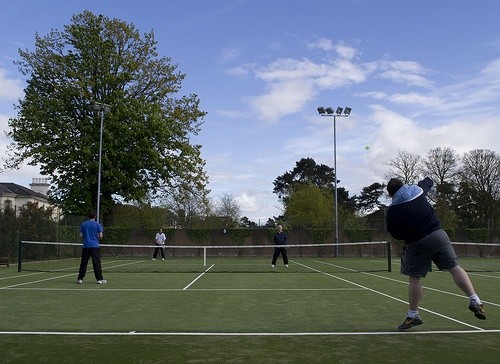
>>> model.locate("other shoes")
[272,265,275,268]
[152,258,156,261]
[78,279,82,284]
[97,279,106,284]
[162,258,164,261]
[285,264,288,267]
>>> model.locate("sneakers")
[469,299,487,320]
[398,315,423,329]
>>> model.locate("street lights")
[317,106,352,258]
[92,101,112,224]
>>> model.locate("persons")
[271,225,289,267]
[386,177,486,329]
[152,228,166,260]
[76,210,107,284]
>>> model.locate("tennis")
[365,146,369,149]
